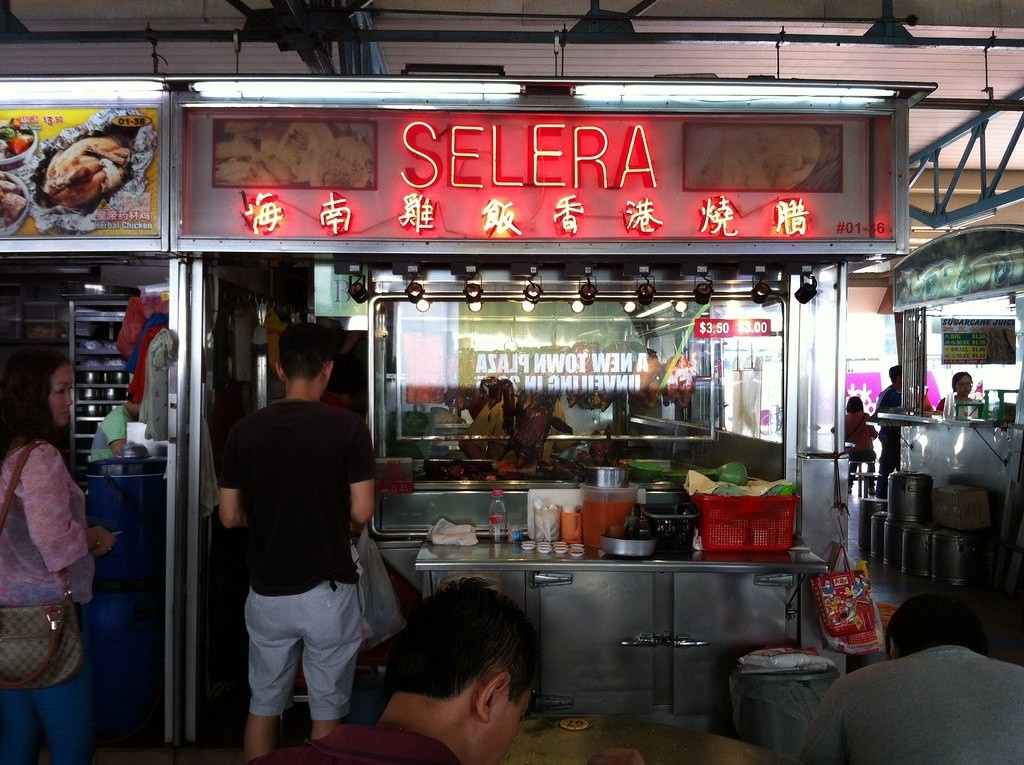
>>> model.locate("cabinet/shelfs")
[65,299,168,479]
[425,570,801,720]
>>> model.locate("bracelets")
[88,527,112,552]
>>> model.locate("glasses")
[957,382,973,387]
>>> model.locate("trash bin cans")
[729,667,841,757]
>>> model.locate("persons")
[90,393,140,475]
[217,322,377,762]
[246,577,541,765]
[876,365,902,499]
[733,348,760,438]
[0,345,117,765]
[798,590,1024,765]
[936,372,973,411]
[845,397,876,495]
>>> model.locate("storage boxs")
[635,500,701,554]
[24,300,67,319]
[932,481,993,532]
[0,282,37,297]
[0,321,23,340]
[0,296,37,322]
[691,492,802,553]
[24,318,68,338]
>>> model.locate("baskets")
[694,493,801,550]
[640,503,699,541]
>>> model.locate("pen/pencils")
[110,531,124,535]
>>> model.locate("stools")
[849,460,877,498]
[849,471,883,498]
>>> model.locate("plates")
[781,127,820,190]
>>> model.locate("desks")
[495,709,815,765]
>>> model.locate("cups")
[508,525,522,543]
[624,515,649,540]
[521,539,585,557]
[533,505,560,542]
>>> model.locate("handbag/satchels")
[353,525,405,651]
[0,598,82,690]
[812,545,885,655]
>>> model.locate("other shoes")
[848,487,851,492]
[869,489,876,494]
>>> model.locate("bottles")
[489,490,506,543]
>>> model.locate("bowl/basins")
[0,124,38,173]
[117,444,150,458]
[79,371,128,383]
[627,460,662,483]
[599,533,657,556]
[661,470,688,484]
[0,170,29,237]
[582,464,632,488]
[82,403,121,417]
[699,468,721,482]
[79,388,127,400]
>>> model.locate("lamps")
[0,75,171,104]
[450,263,485,303]
[620,302,636,313]
[563,262,599,307]
[566,78,900,107]
[568,301,585,313]
[736,263,772,306]
[680,263,715,307]
[0,264,91,273]
[467,302,483,312]
[390,261,427,304]
[520,301,536,313]
[508,262,545,304]
[629,264,656,304]
[416,299,431,313]
[332,261,370,305]
[670,301,688,314]
[186,78,530,107]
[787,265,819,305]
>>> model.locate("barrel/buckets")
[83,458,166,746]
[858,472,973,586]
[676,377,719,423]
[579,482,639,548]
